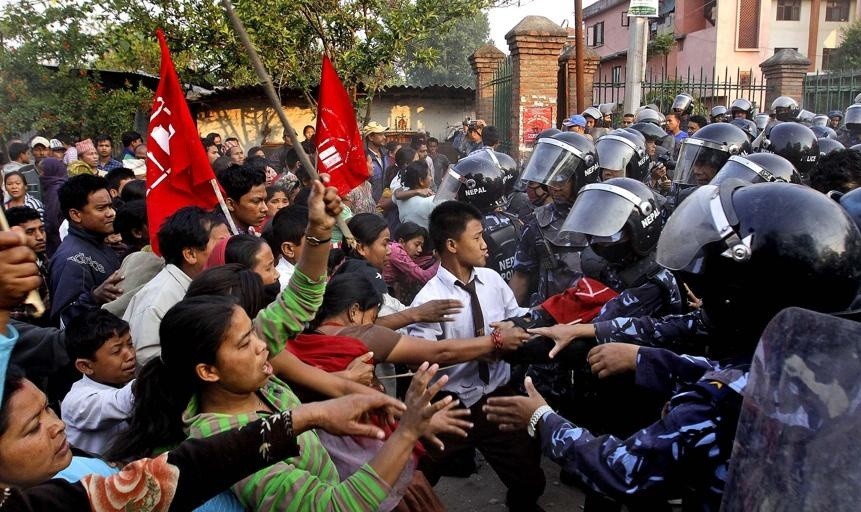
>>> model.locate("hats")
[221,141,238,154]
[31,137,96,155]
[283,128,297,136]
[364,121,389,137]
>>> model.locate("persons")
[0,93,861,511]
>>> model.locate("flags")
[315,49,370,198]
[144,28,226,257]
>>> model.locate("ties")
[454,280,489,384]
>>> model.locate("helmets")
[493,150,519,193]
[525,95,861,359]
[452,150,505,209]
